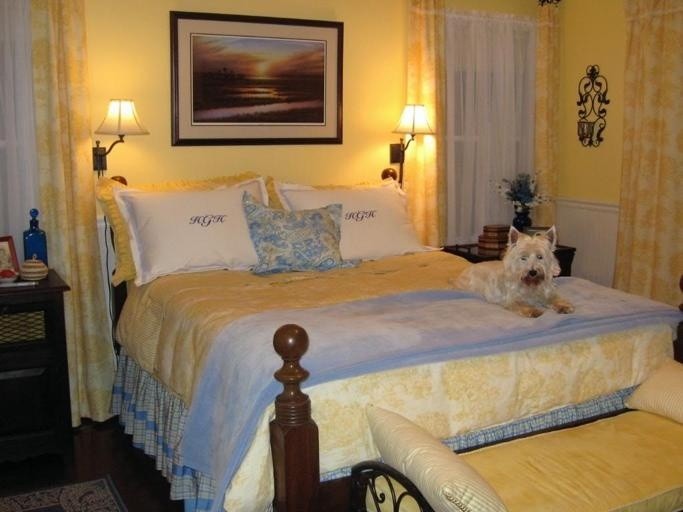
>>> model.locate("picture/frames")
[168,9,344,146]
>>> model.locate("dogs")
[452,224,575,318]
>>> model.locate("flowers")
[493,173,550,212]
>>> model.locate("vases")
[512,211,533,231]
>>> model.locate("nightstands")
[0,267,78,498]
[441,244,577,277]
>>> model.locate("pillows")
[624,360,682,422]
[114,176,269,287]
[241,190,353,275]
[274,180,423,264]
[96,170,281,290]
[365,401,508,512]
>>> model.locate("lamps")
[93,98,150,171]
[576,64,610,148]
[390,103,435,164]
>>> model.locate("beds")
[95,168,683,511]
[350,357,683,511]
